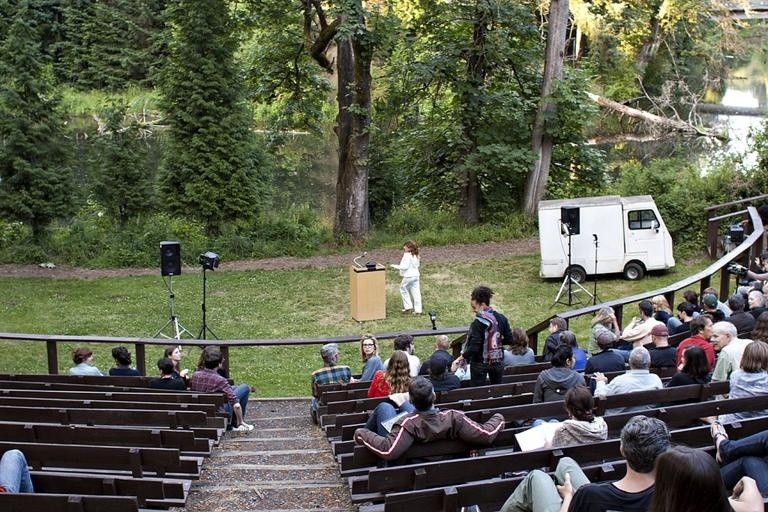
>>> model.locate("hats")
[320,344,340,359]
[594,330,614,344]
[648,324,669,336]
[702,295,717,308]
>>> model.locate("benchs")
[309,314,767,512]
[0,366,240,512]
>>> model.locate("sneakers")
[232,424,253,432]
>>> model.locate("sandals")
[711,420,730,465]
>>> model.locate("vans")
[532,197,676,286]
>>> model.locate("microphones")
[353,251,367,268]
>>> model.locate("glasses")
[363,344,373,348]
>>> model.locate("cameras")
[726,265,748,278]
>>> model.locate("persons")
[389,240,423,315]
[710,419,768,496]
[358,249,767,436]
[646,444,734,511]
[726,475,765,511]
[351,375,507,461]
[349,334,384,382]
[65,342,256,432]
[499,414,672,511]
[531,383,609,448]
[310,342,351,425]
[0,447,36,493]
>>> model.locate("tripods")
[549,245,594,308]
[151,283,196,352]
[187,274,219,354]
[577,255,605,317]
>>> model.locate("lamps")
[551,218,586,312]
[192,249,223,351]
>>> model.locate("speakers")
[160,240,182,276]
[560,206,581,235]
